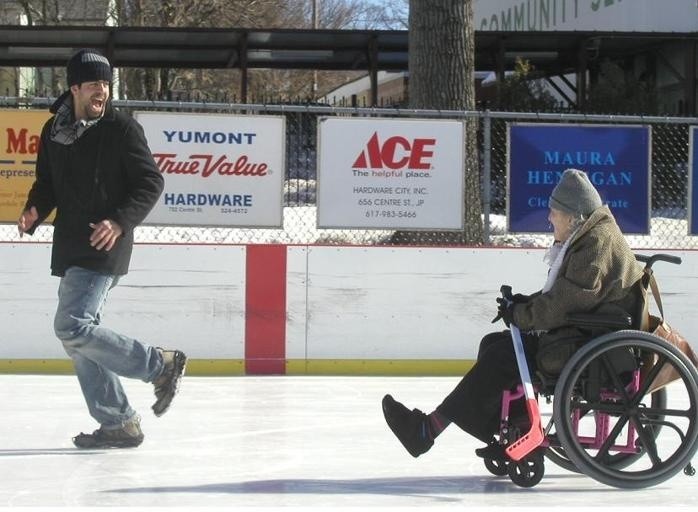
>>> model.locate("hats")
[66,44,113,88]
[547,167,604,217]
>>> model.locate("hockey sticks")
[498,286,545,461]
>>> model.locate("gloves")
[491,285,526,330]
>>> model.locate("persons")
[16,45,186,453]
[380,167,643,461]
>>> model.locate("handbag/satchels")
[639,268,698,395]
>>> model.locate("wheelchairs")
[484,253,698,490]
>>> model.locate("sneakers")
[473,435,530,459]
[150,350,188,418]
[382,393,435,459]
[71,418,145,450]
